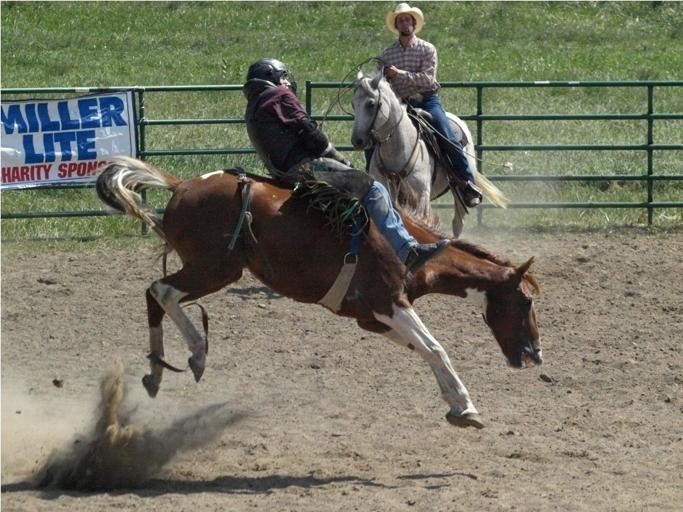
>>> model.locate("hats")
[386,3,424,36]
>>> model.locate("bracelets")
[324,145,348,165]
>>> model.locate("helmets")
[247,58,288,86]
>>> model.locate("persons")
[364,2,484,208]
[242,56,452,275]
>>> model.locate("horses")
[350,65,510,239]
[94,155,543,429]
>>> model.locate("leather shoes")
[464,184,482,207]
[405,238,452,275]
